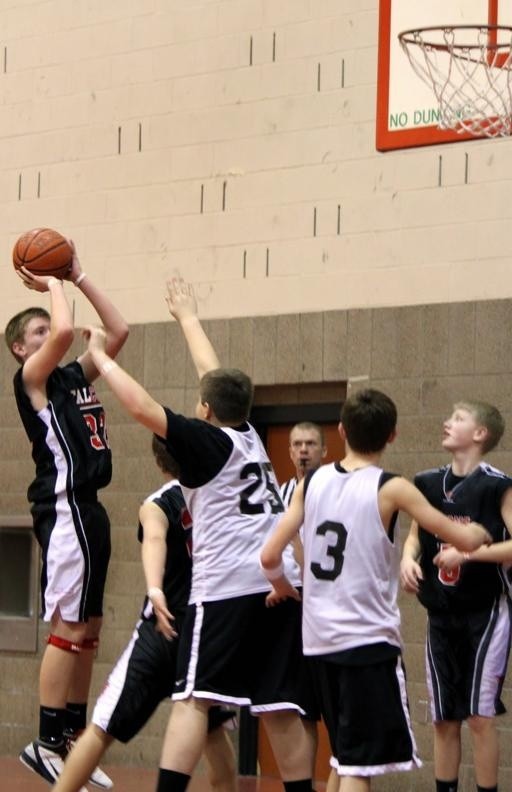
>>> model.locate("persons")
[52,431,239,792]
[82,276,316,792]
[6,240,118,791]
[258,388,493,789]
[398,400,512,791]
[277,419,341,790]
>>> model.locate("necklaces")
[437,462,481,505]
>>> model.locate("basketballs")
[11,227,72,279]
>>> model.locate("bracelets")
[74,272,87,287]
[258,559,284,580]
[46,278,63,287]
[147,586,163,598]
[99,362,118,376]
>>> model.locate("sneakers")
[18,739,87,792]
[64,730,115,790]
